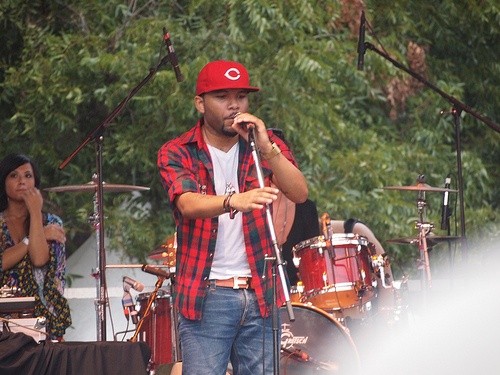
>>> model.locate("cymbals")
[43,180,152,192]
[381,183,458,193]
[385,232,462,246]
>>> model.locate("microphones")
[357,11,366,71]
[163,28,185,82]
[123,277,144,292]
[141,265,170,279]
[319,360,340,375]
[440,175,451,230]
[234,112,255,131]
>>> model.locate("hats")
[196,60,259,96]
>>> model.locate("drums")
[291,232,377,312]
[225,302,359,375]
[135,292,181,370]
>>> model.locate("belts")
[214,276,252,291]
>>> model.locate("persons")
[318,218,394,286]
[267,130,321,289]
[158,60,308,375]
[0,153,72,338]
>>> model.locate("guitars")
[162,236,182,375]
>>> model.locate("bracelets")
[22,236,29,245]
[259,141,281,162]
[222,194,239,220]
